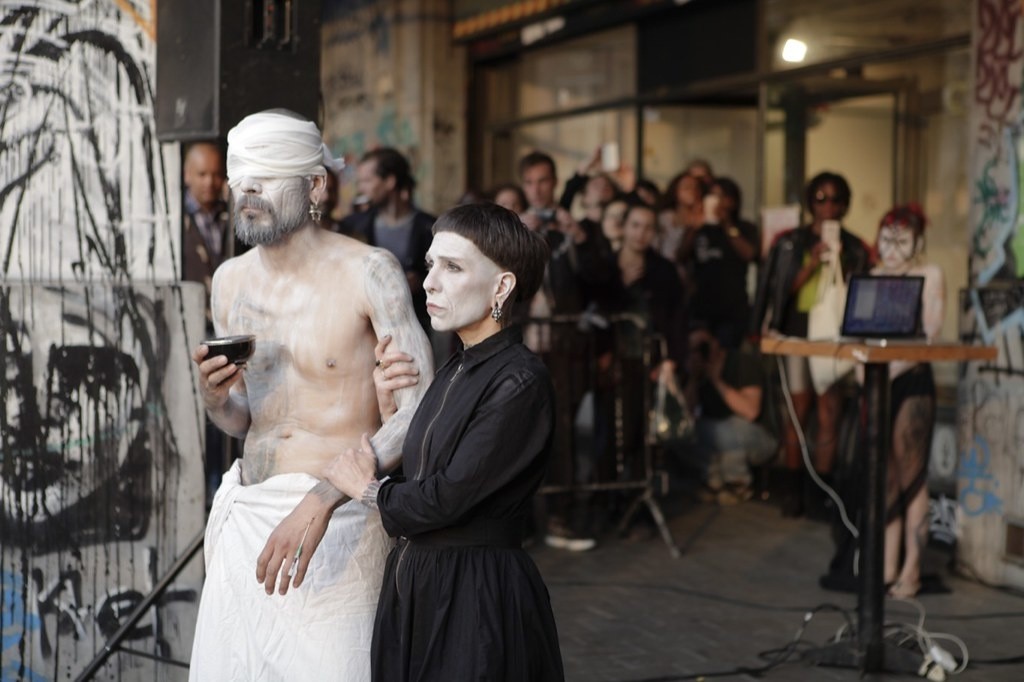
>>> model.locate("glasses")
[815,192,840,206]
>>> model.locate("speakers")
[155,0,321,145]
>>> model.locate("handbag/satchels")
[644,361,703,453]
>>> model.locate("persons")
[319,202,564,682]
[857,203,942,598]
[180,137,869,522]
[189,108,434,682]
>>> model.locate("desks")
[761,335,998,682]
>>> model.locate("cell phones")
[600,142,619,172]
[703,195,720,225]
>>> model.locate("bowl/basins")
[200,334,255,370]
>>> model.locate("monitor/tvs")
[840,275,925,338]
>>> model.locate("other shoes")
[543,519,597,550]
[618,522,653,544]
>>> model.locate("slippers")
[883,577,921,601]
